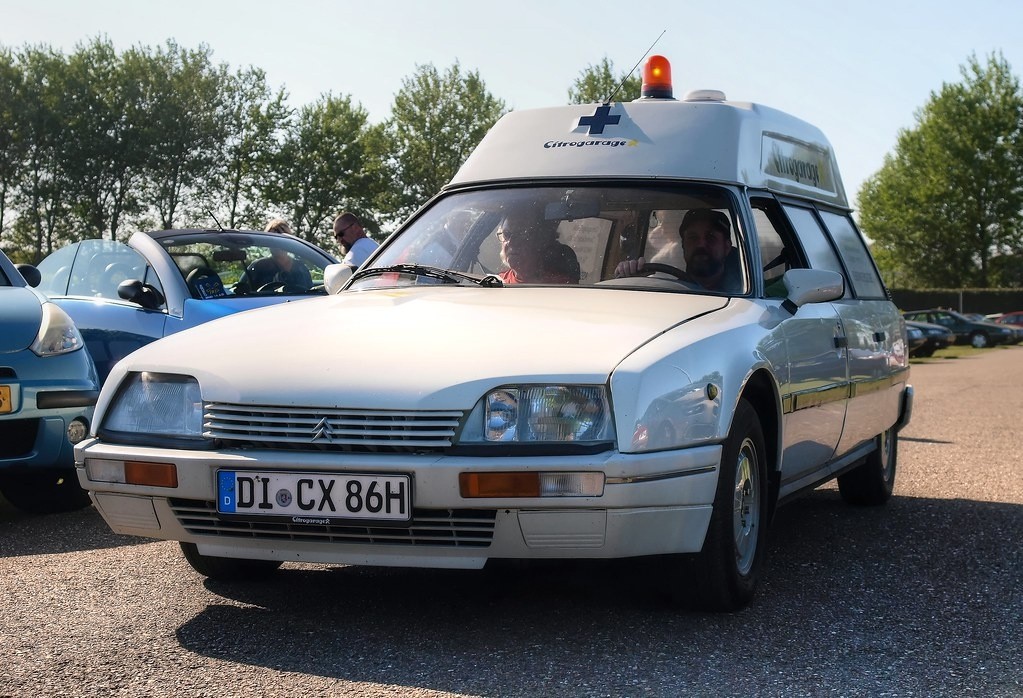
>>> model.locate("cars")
[0,249,101,517]
[903,309,1018,349]
[907,319,956,359]
[941,311,1023,346]
[32,226,359,389]
[985,312,1004,323]
[997,310,1023,326]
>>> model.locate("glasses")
[496,229,544,243]
[334,223,354,240]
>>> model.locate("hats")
[679,209,731,240]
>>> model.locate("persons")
[614,208,742,295]
[493,198,565,284]
[332,213,380,272]
[240,218,313,295]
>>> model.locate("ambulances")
[74,55,912,615]
